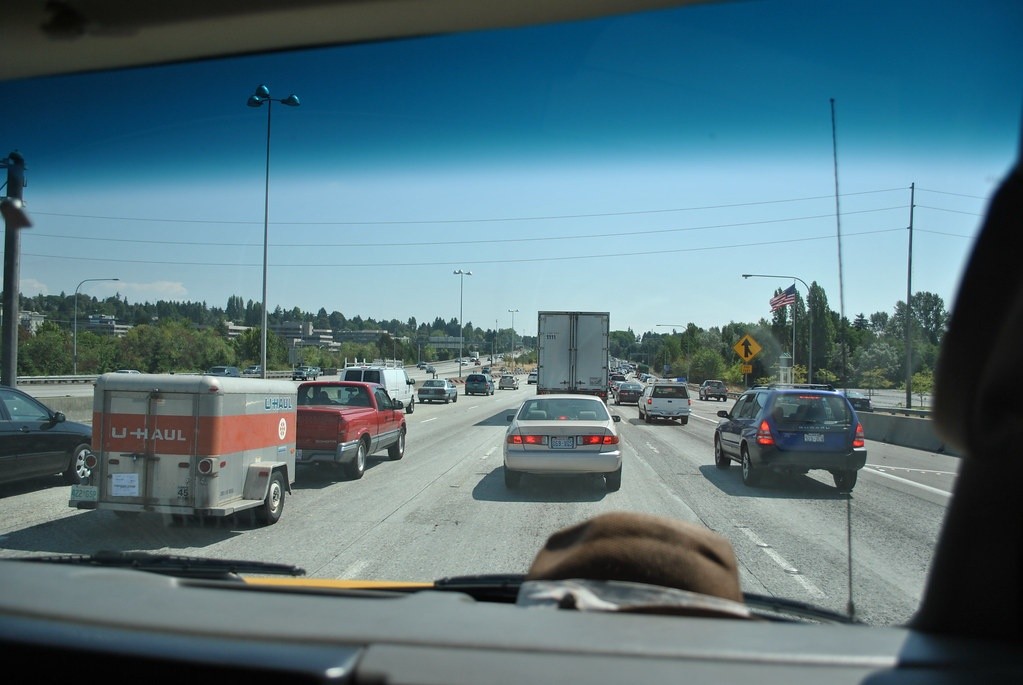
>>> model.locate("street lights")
[74,278,119,374]
[508,309,518,372]
[743,274,812,382]
[454,270,472,377]
[656,324,689,380]
[248,84,300,379]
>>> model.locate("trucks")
[537,310,611,404]
[470,351,479,359]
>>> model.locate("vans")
[464,375,494,396]
[68,366,297,523]
[338,366,415,414]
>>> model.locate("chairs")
[310,391,332,405]
[796,405,822,423]
[577,410,598,420]
[528,410,547,421]
[346,390,370,407]
[772,406,789,423]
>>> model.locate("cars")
[608,358,644,405]
[0,385,92,495]
[503,393,622,489]
[417,354,499,374]
[418,380,457,404]
[840,392,874,412]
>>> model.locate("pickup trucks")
[295,382,407,479]
[293,366,318,381]
[699,380,727,402]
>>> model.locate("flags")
[769,283,795,313]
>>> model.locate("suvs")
[639,381,691,423]
[714,383,867,489]
[499,371,537,390]
